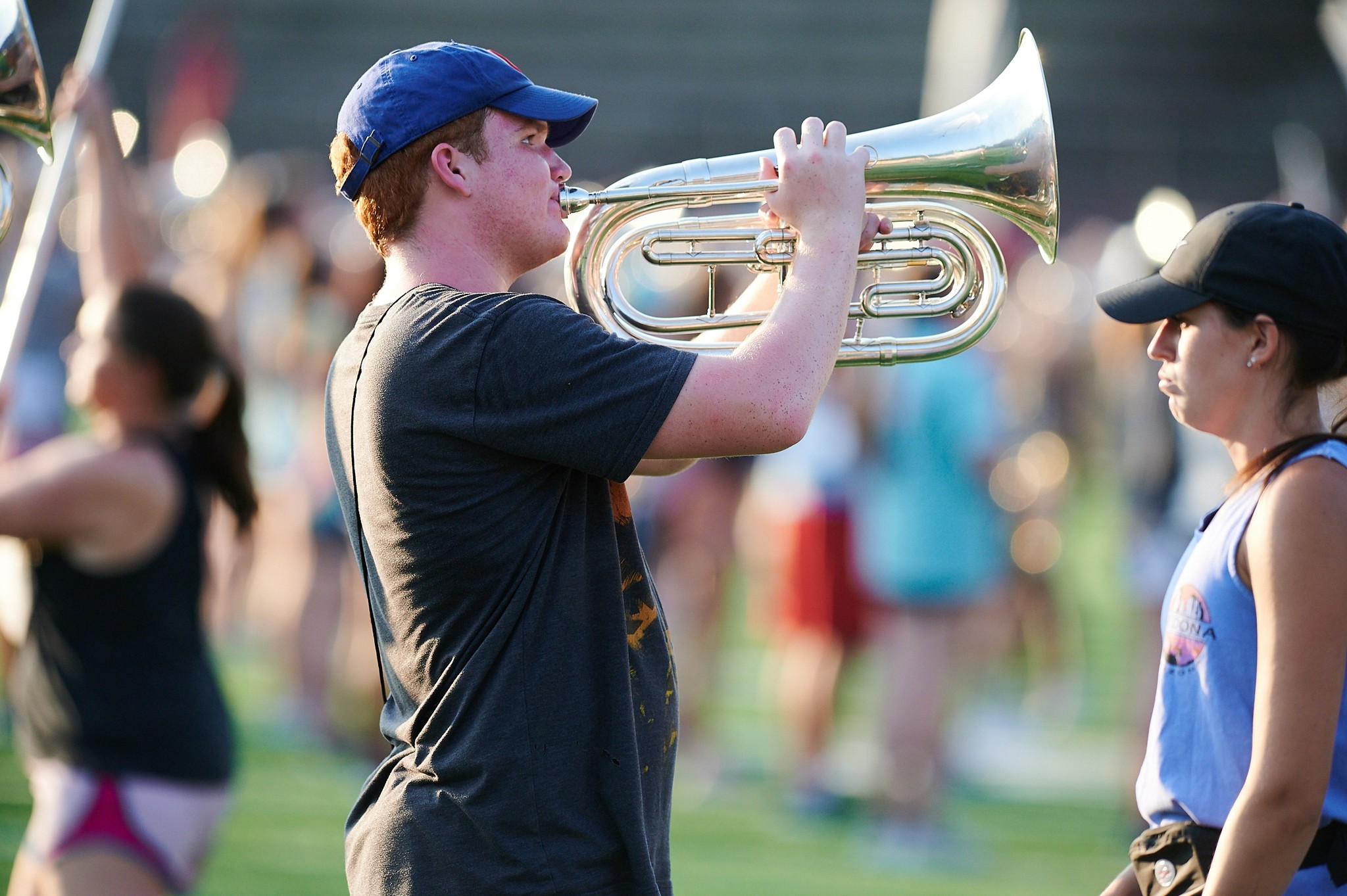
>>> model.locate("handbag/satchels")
[1128,821,1212,896]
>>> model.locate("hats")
[1097,202,1347,338]
[336,42,598,202]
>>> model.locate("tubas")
[558,26,1059,365]
[0,2,55,242]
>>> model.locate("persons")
[330,38,889,896]
[1095,197,1346,896]
[0,62,262,896]
[0,177,1235,873]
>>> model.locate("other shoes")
[843,804,998,873]
